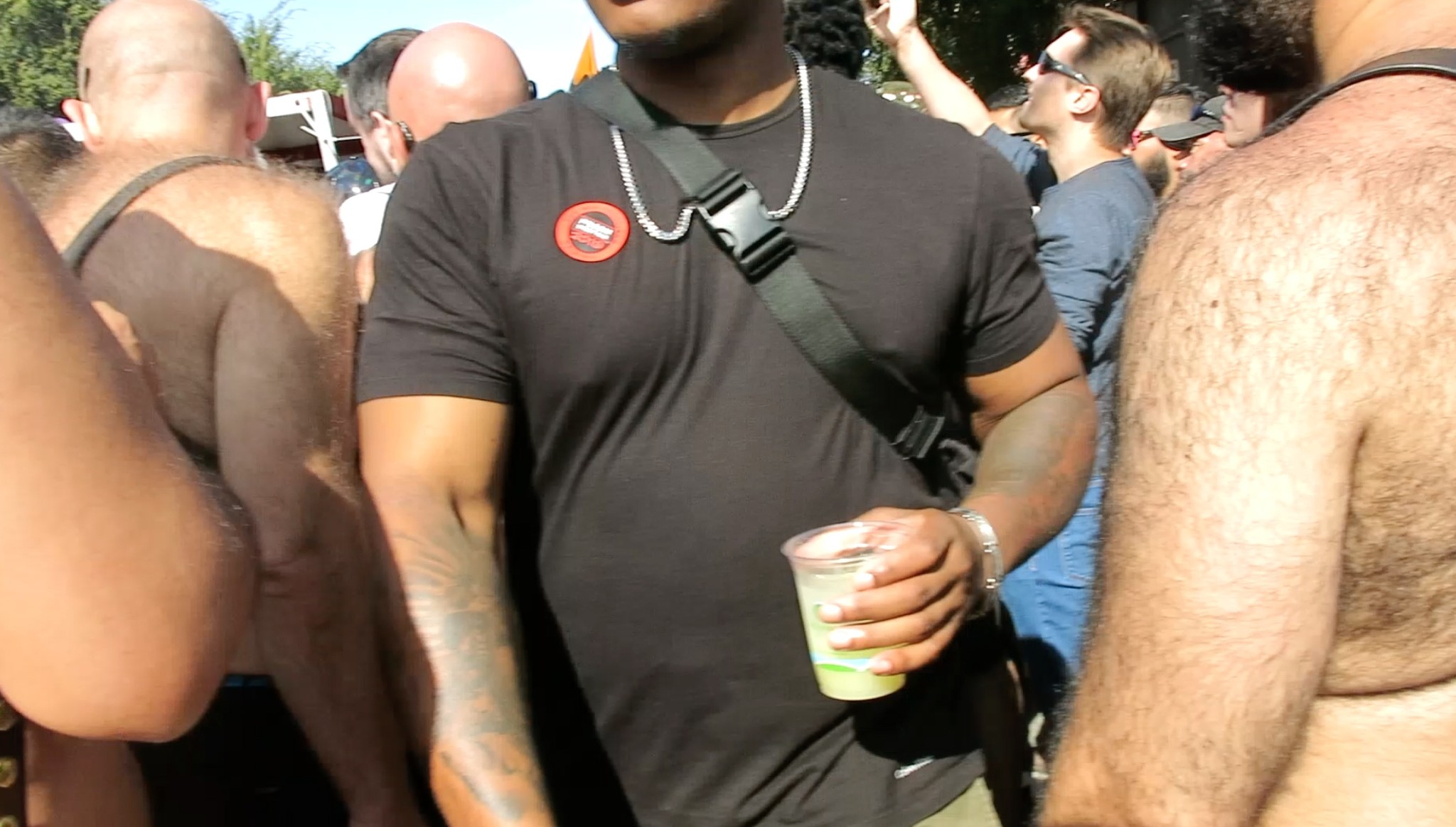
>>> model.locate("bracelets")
[948,505,1006,626]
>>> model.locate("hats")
[1152,93,1232,144]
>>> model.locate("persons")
[27,1,433,827]
[0,100,80,194]
[1030,0,1456,827]
[855,0,1321,690]
[1,166,261,748]
[334,19,528,303]
[350,0,1102,826]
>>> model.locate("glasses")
[1131,130,1152,150]
[1037,50,1107,108]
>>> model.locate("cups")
[781,521,905,702]
